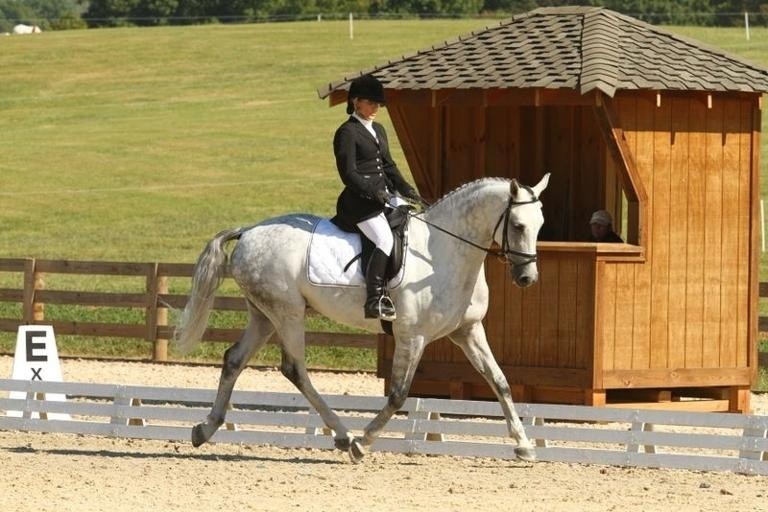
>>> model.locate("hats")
[589,210,612,226]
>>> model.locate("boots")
[364,247,394,319]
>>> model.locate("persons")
[332,73,422,321]
[588,209,622,243]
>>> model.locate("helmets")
[346,73,385,115]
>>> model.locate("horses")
[160,172,550,465]
